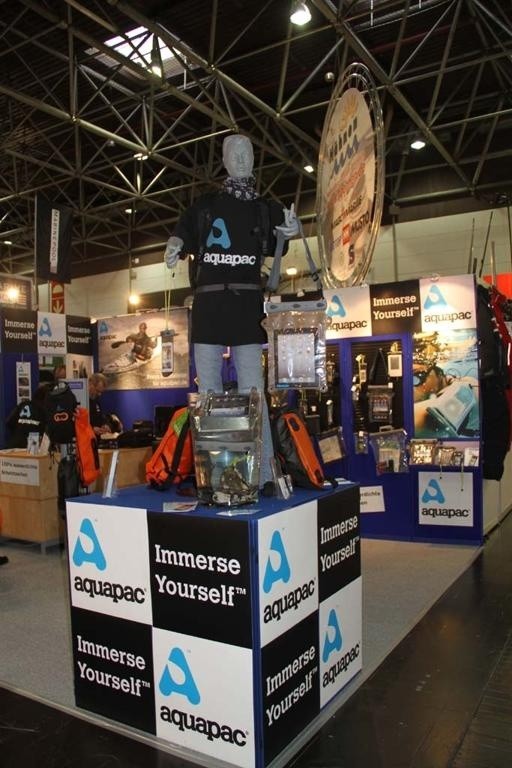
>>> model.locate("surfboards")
[102,349,155,374]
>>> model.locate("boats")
[100,339,164,375]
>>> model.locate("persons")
[125,323,158,361]
[409,360,479,434]
[86,375,125,438]
[159,135,302,497]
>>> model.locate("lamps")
[289,0,312,26]
[150,36,163,76]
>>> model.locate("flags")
[34,194,75,286]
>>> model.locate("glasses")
[412,363,435,387]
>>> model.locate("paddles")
[111,334,180,349]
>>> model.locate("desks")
[0,447,153,555]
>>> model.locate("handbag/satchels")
[273,407,326,491]
[361,350,399,427]
[57,455,88,519]
[264,273,329,393]
[74,406,101,488]
[369,425,410,475]
[145,406,193,489]
